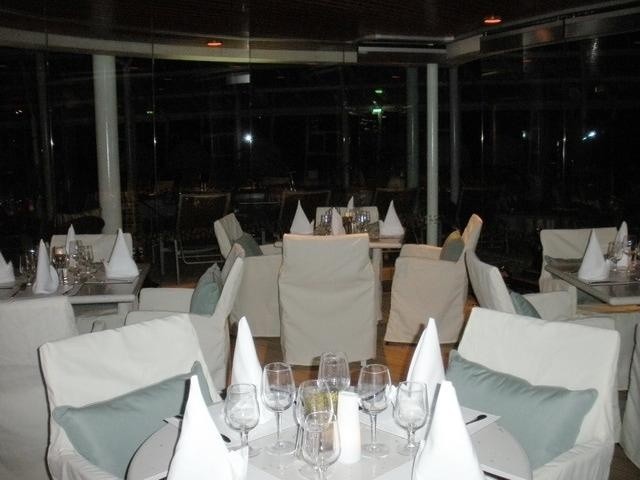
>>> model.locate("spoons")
[6,281,39,297]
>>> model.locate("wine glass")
[296,380,334,463]
[262,362,296,454]
[317,352,351,408]
[51,245,70,295]
[77,244,94,285]
[19,254,37,296]
[392,382,428,457]
[357,364,392,458]
[224,383,260,458]
[622,238,638,276]
[302,412,340,480]
[609,242,624,274]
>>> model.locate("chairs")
[157,192,232,284]
[0,295,81,479]
[538,227,618,291]
[312,204,380,227]
[125,242,246,392]
[278,189,331,235]
[46,234,133,264]
[39,311,222,480]
[0,252,16,283]
[465,249,616,330]
[452,307,623,480]
[277,232,379,368]
[387,211,484,342]
[214,212,281,337]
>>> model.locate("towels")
[616,221,629,249]
[405,317,446,395]
[231,316,267,399]
[64,223,78,254]
[577,228,609,280]
[166,375,241,480]
[289,199,314,236]
[330,206,346,236]
[104,227,139,278]
[0,250,14,282]
[345,195,355,211]
[33,239,59,295]
[381,200,405,235]
[412,381,484,480]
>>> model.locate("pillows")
[446,349,597,469]
[510,289,542,319]
[51,361,212,479]
[235,231,263,256]
[440,228,466,261]
[187,260,223,315]
